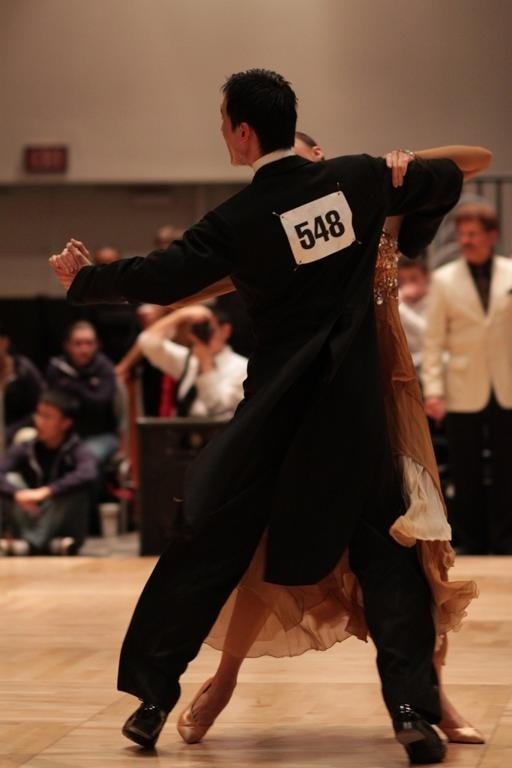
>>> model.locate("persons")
[138,227,217,419]
[0,323,127,556]
[50,134,494,745]
[49,66,463,768]
[395,204,512,555]
[138,303,248,422]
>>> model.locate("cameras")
[192,321,215,345]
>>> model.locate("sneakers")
[0,535,80,555]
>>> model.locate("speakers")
[136,418,230,557]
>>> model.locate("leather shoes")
[392,701,446,763]
[435,707,485,745]
[178,673,219,745]
[121,700,167,749]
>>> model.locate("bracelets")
[403,147,420,161]
